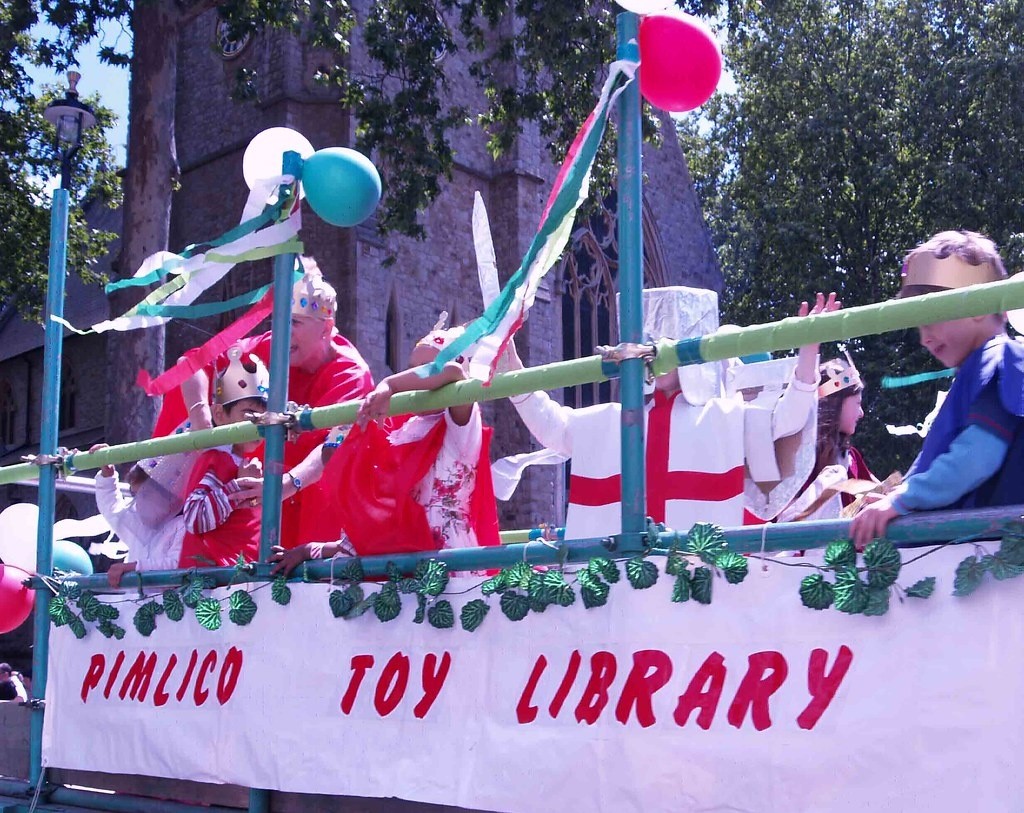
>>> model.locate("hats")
[613,286,722,408]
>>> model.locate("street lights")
[41,69,99,189]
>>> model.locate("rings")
[250,499,258,506]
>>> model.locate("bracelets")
[189,400,209,411]
[310,542,325,560]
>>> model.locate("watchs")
[287,471,302,493]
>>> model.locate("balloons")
[1006,272,1024,336]
[638,15,721,112]
[0,503,39,574]
[0,562,38,634]
[301,147,383,227]
[53,541,93,575]
[615,0,679,13]
[242,126,315,206]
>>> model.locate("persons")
[477,283,843,539]
[847,230,1024,550]
[89,438,187,595]
[719,320,906,524]
[182,349,264,535]
[320,312,488,581]
[176,267,374,512]
[0,662,32,703]
